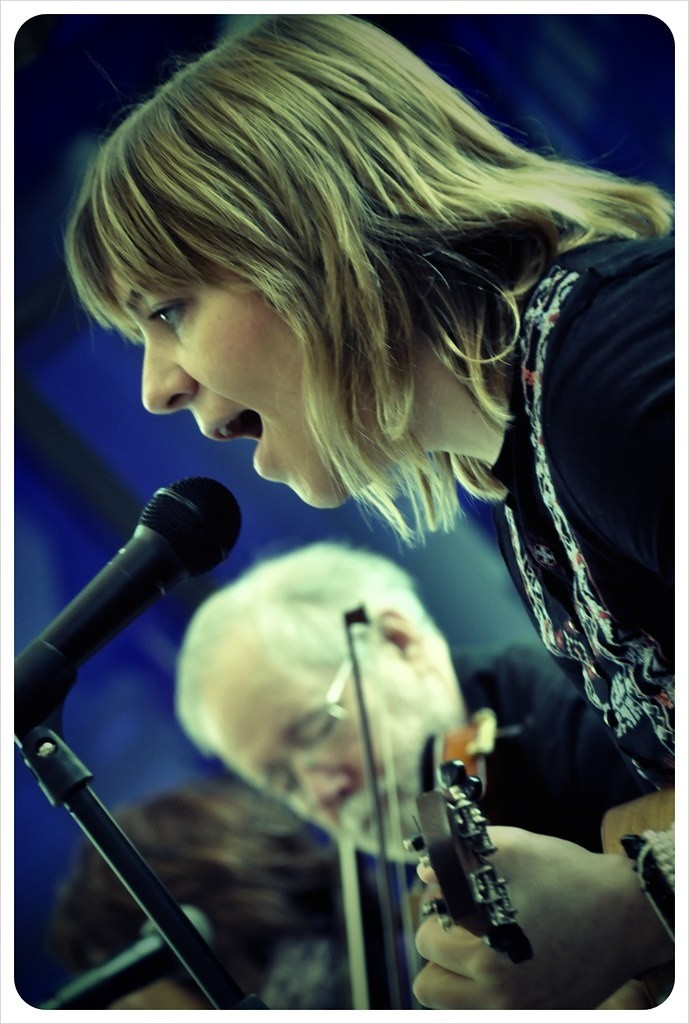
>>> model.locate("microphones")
[39,906,213,1012]
[16,477,244,737]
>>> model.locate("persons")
[56,14,674,1010]
[172,546,656,855]
[52,781,420,1011]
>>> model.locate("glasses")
[240,632,378,820]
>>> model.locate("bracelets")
[618,823,674,942]
